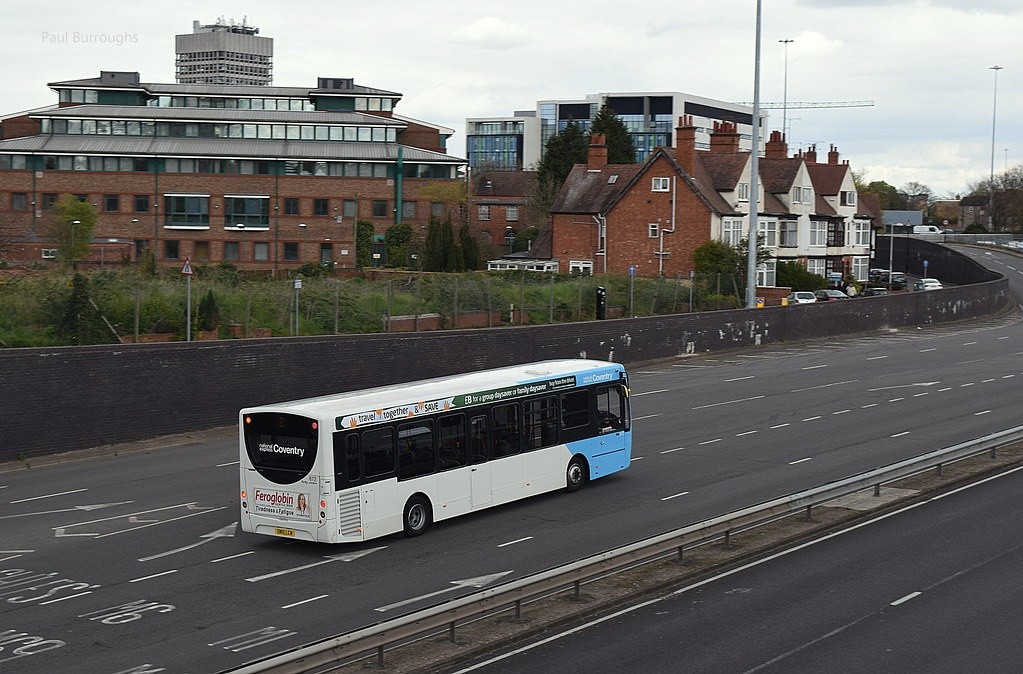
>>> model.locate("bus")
[238,357,633,544]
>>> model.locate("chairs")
[372,440,508,470]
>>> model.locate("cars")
[813,289,849,303]
[913,279,943,291]
[870,268,908,286]
[786,292,817,304]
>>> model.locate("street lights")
[988,65,1003,228]
[780,39,793,143]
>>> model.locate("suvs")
[855,288,890,298]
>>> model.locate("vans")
[914,225,941,233]
